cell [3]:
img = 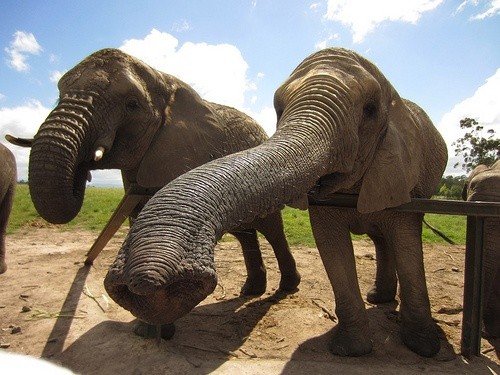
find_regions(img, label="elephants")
[3,48,302,338]
[103,47,458,361]
[0,143,18,274]
